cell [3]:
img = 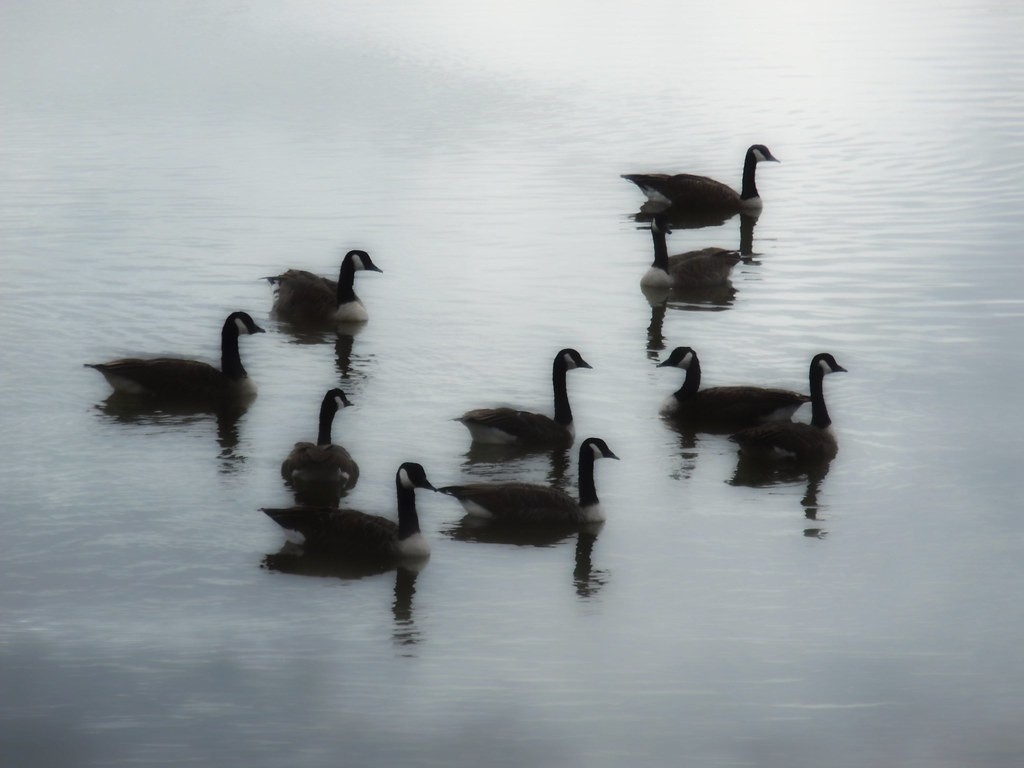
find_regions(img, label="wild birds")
[282,388,360,490]
[436,437,620,523]
[640,216,745,291]
[727,353,849,462]
[450,348,593,446]
[619,144,781,217]
[259,250,384,323]
[655,346,813,424]
[256,462,439,562]
[83,311,267,401]
